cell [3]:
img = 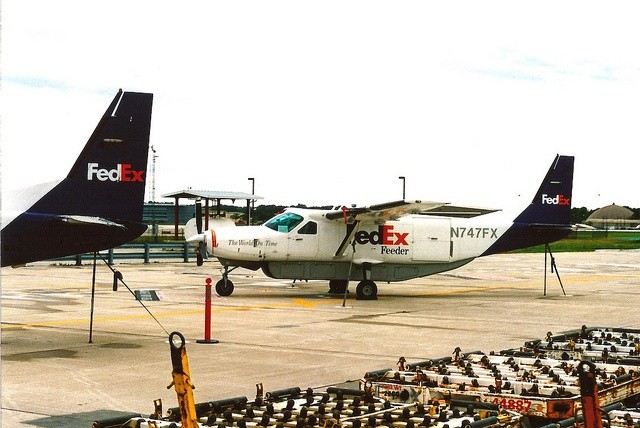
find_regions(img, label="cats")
[183,154,574,301]
[1,88,154,270]
